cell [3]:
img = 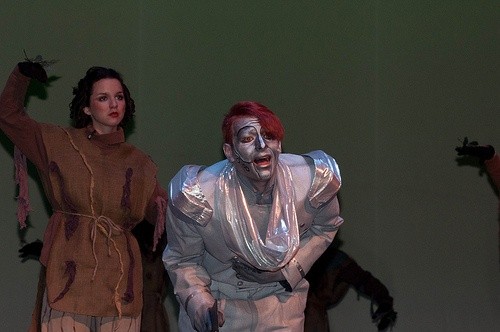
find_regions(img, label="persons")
[0,46,168,332]
[163,101,342,332]
